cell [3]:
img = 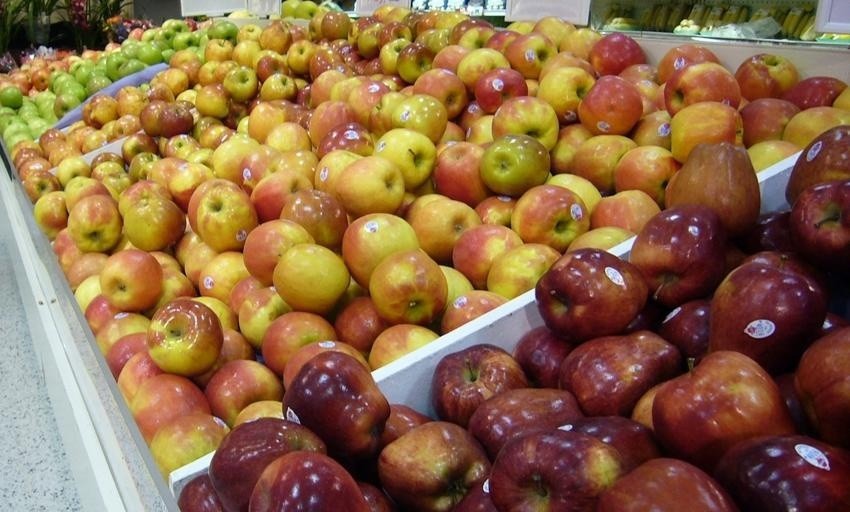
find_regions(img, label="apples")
[1,1,849,512]
